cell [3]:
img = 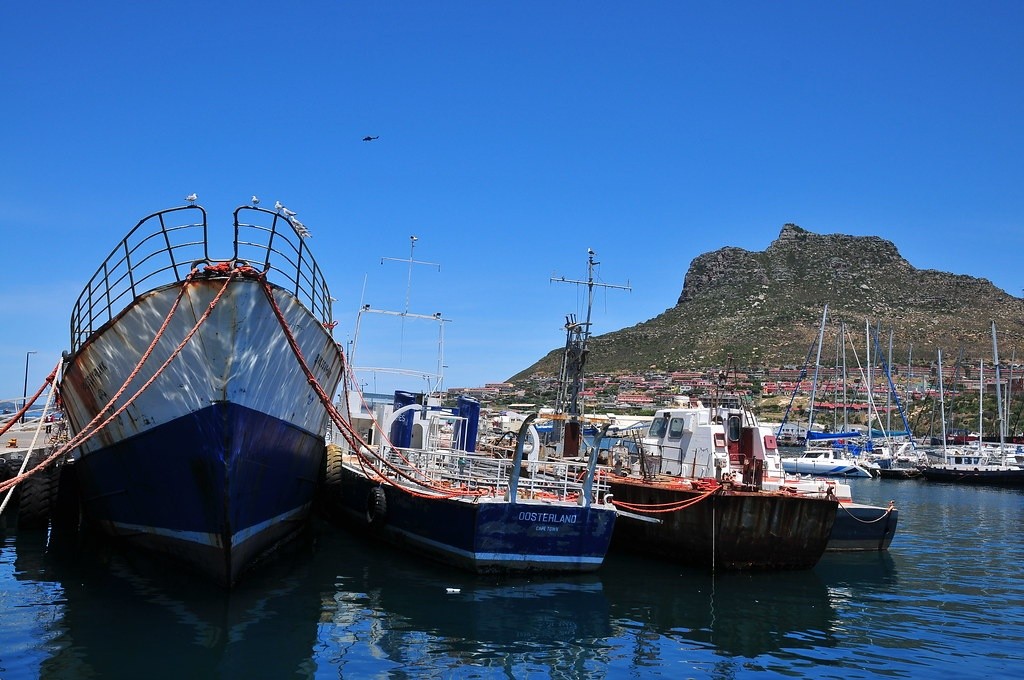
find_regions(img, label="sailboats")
[0,191,1024,565]
